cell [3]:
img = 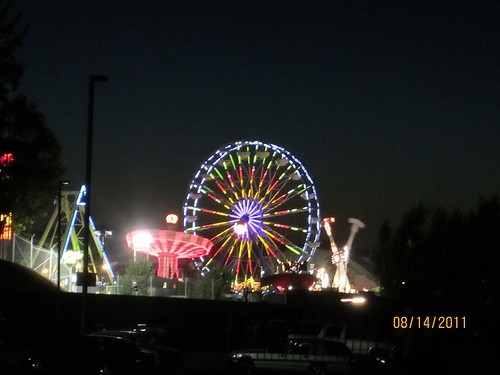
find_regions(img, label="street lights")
[81,75,107,294]
[57,180,70,287]
